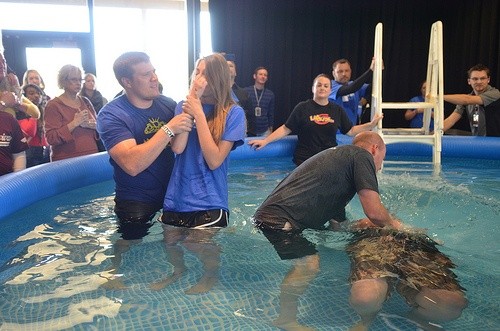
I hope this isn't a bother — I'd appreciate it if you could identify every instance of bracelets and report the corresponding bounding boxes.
[415,108,419,115]
[160,124,176,141]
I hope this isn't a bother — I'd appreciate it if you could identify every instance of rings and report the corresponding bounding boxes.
[88,120,91,125]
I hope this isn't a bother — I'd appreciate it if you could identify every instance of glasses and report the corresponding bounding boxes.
[64,78,85,82]
[469,76,489,81]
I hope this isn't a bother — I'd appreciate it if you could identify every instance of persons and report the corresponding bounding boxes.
[248,72,384,167]
[242,65,275,137]
[404,63,500,138]
[328,57,384,135]
[252,130,471,331]
[0,50,247,296]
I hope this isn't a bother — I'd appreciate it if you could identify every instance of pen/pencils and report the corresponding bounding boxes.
[78,108,81,113]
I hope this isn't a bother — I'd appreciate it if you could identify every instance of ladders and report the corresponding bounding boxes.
[370,21,444,174]
